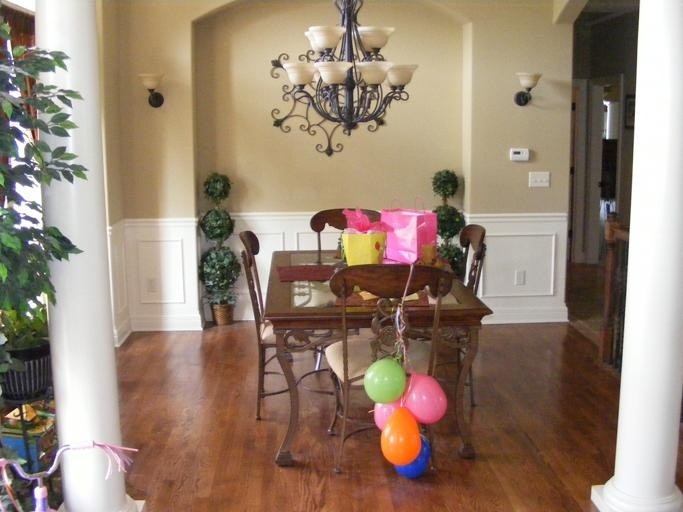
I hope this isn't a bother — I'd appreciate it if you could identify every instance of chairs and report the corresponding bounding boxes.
[235,226,341,423]
[401,223,487,410]
[320,259,454,479]
[308,208,385,376]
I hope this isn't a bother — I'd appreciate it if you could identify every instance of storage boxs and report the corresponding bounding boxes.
[0,414,59,484]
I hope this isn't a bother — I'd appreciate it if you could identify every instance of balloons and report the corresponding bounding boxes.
[363,357,447,477]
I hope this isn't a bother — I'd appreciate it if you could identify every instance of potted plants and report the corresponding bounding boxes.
[0,15,92,406]
[195,168,240,326]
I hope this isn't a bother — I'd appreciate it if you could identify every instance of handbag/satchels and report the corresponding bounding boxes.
[377,194,440,264]
[341,226,388,267]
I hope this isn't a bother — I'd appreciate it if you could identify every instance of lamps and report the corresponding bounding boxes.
[135,69,167,111]
[509,70,544,107]
[277,1,426,138]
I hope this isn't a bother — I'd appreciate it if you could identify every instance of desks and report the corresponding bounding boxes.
[261,245,494,470]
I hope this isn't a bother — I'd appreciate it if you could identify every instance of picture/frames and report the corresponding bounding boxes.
[622,92,635,130]
